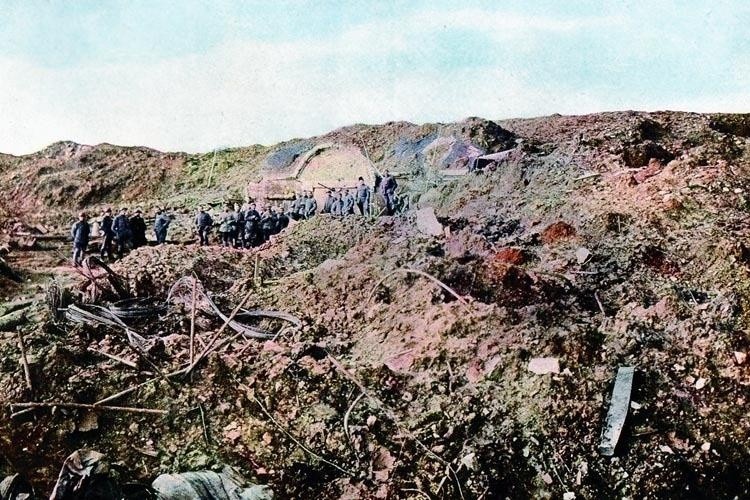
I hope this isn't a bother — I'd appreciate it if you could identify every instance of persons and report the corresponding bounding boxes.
[379,169,398,216]
[154,206,170,244]
[98,207,148,263]
[193,177,371,250]
[70,212,90,267]
